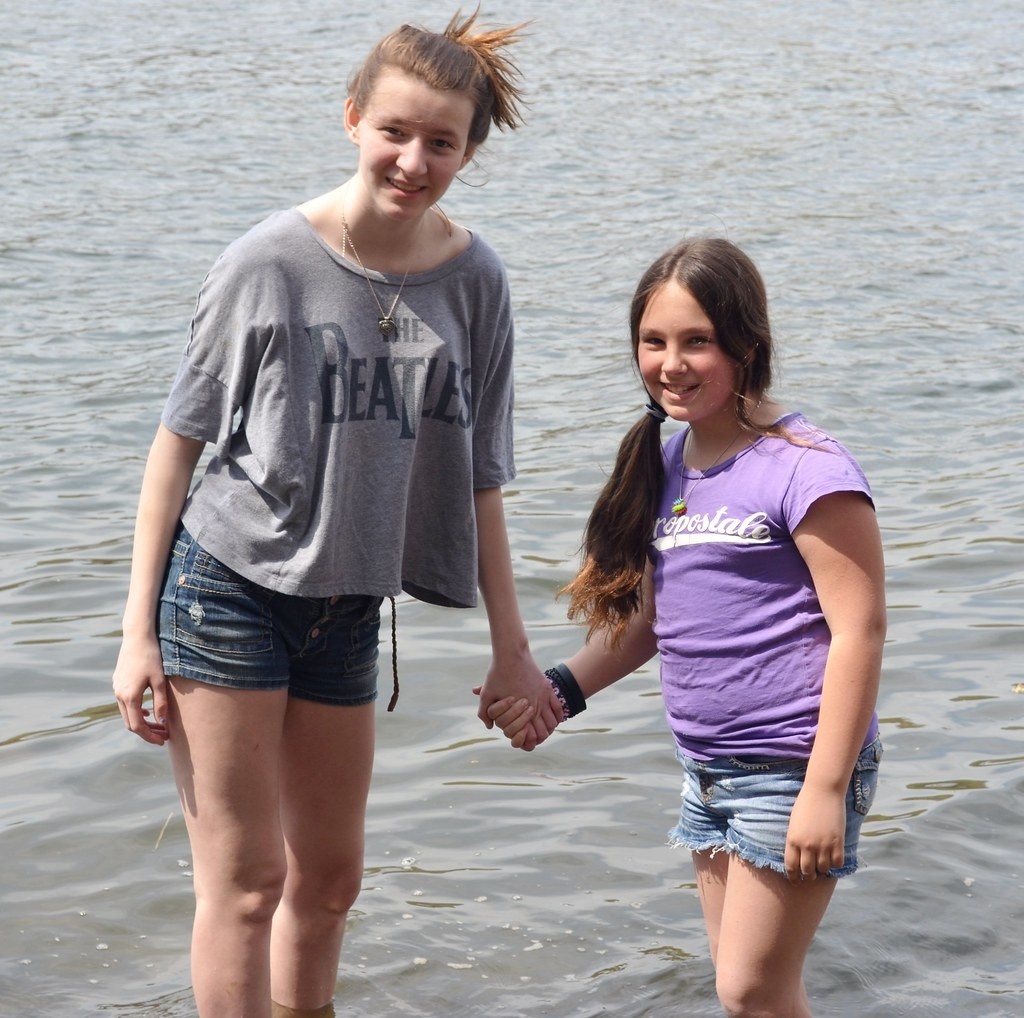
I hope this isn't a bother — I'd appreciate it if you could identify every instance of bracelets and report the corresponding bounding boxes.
[542,664,587,721]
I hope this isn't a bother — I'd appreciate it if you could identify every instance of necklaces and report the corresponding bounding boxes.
[339,185,427,336]
[670,398,765,514]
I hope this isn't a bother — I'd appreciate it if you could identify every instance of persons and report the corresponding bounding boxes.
[473,234,888,1017]
[112,9,565,1018]
